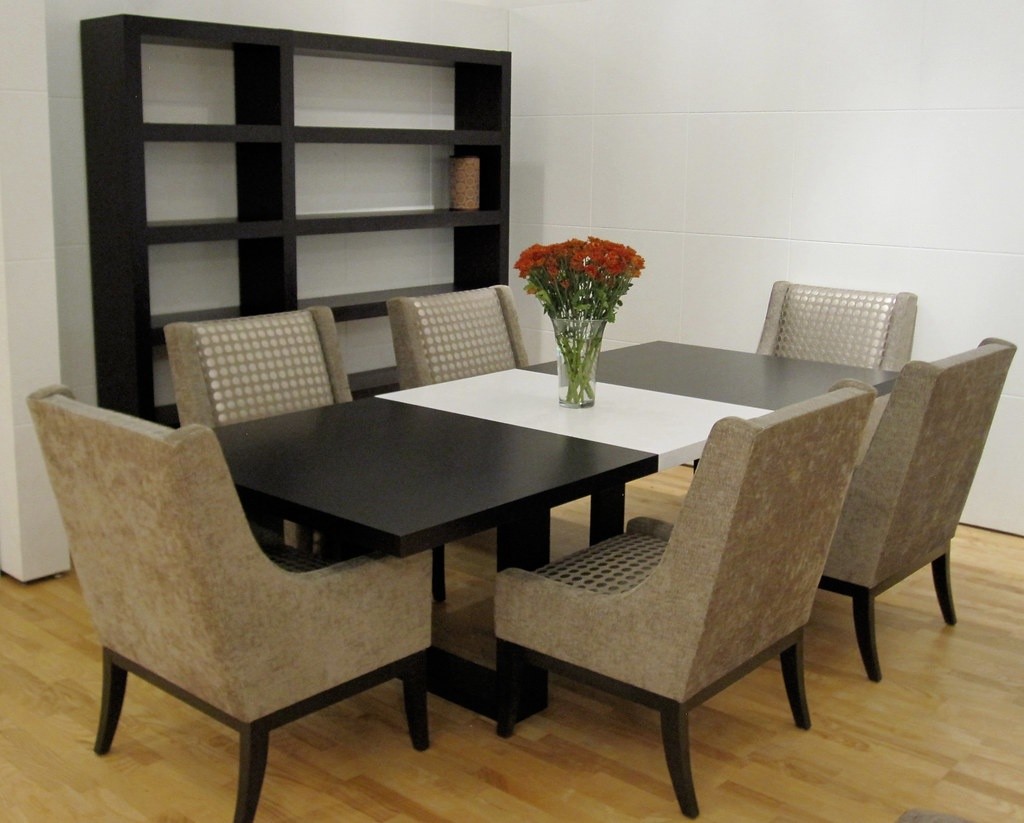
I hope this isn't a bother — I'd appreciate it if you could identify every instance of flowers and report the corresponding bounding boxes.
[513,235,646,404]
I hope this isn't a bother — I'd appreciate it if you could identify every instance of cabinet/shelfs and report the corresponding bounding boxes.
[79,13,513,431]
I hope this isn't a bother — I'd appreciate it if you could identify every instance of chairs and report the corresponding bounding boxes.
[818,338,1017,684]
[25,385,434,823]
[492,379,880,820]
[754,280,919,466]
[386,285,533,603]
[162,306,358,573]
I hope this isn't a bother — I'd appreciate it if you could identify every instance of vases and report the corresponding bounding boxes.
[549,318,611,408]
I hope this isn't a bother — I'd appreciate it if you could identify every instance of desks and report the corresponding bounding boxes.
[213,343,908,728]
